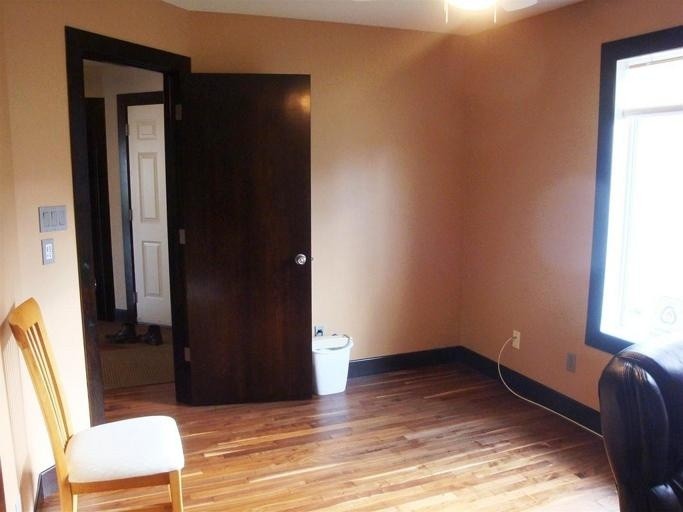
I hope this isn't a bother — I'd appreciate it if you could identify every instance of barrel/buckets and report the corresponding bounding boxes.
[312,334,354,395]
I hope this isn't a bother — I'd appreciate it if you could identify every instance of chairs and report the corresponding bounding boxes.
[7,297,185,512]
[598,339,683,512]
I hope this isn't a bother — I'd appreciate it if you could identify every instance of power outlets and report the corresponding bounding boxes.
[512,330,520,351]
[41,238,55,265]
